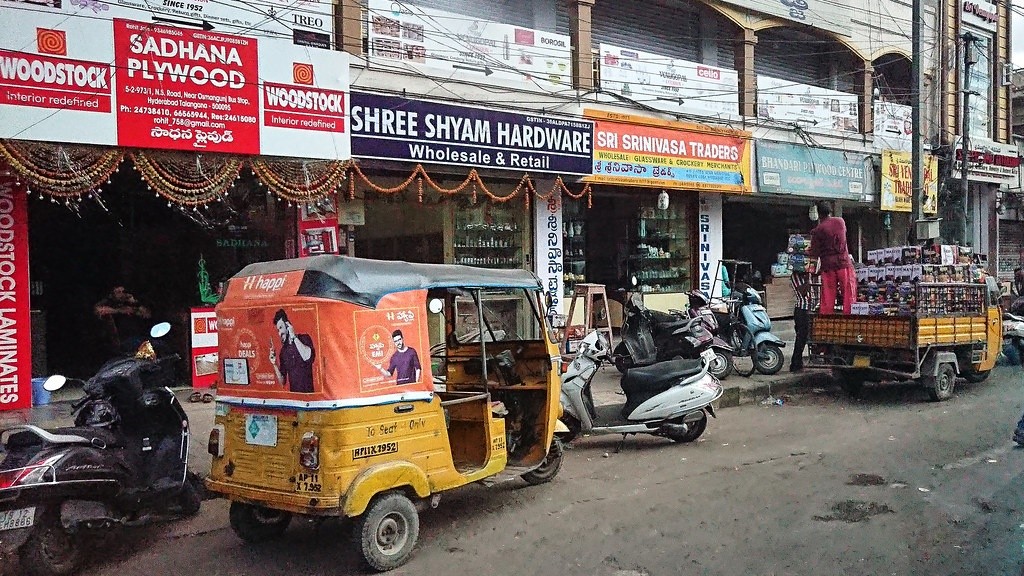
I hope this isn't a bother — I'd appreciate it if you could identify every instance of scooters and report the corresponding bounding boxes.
[553,328,725,453]
[607,273,789,381]
[0,319,203,576]
[1001,311,1024,364]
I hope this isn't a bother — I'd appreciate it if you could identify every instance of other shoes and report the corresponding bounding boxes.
[791,368,813,374]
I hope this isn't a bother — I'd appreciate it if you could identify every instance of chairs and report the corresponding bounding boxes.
[100,311,137,358]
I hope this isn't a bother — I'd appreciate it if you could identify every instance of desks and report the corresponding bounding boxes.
[558,282,614,356]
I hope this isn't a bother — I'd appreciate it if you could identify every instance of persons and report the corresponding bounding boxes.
[790,271,821,372]
[94,283,146,356]
[375,330,421,385]
[808,202,858,315]
[1012,268,1024,316]
[269,309,315,393]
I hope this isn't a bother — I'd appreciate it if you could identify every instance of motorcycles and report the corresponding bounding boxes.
[200,254,571,572]
[800,276,1007,401]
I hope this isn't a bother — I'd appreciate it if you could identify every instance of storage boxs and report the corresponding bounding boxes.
[847,244,986,317]
[770,233,822,277]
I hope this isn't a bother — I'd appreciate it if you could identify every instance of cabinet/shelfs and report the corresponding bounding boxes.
[637,200,689,295]
[561,185,587,297]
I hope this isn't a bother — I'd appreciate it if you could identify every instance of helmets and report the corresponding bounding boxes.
[75,400,121,435]
